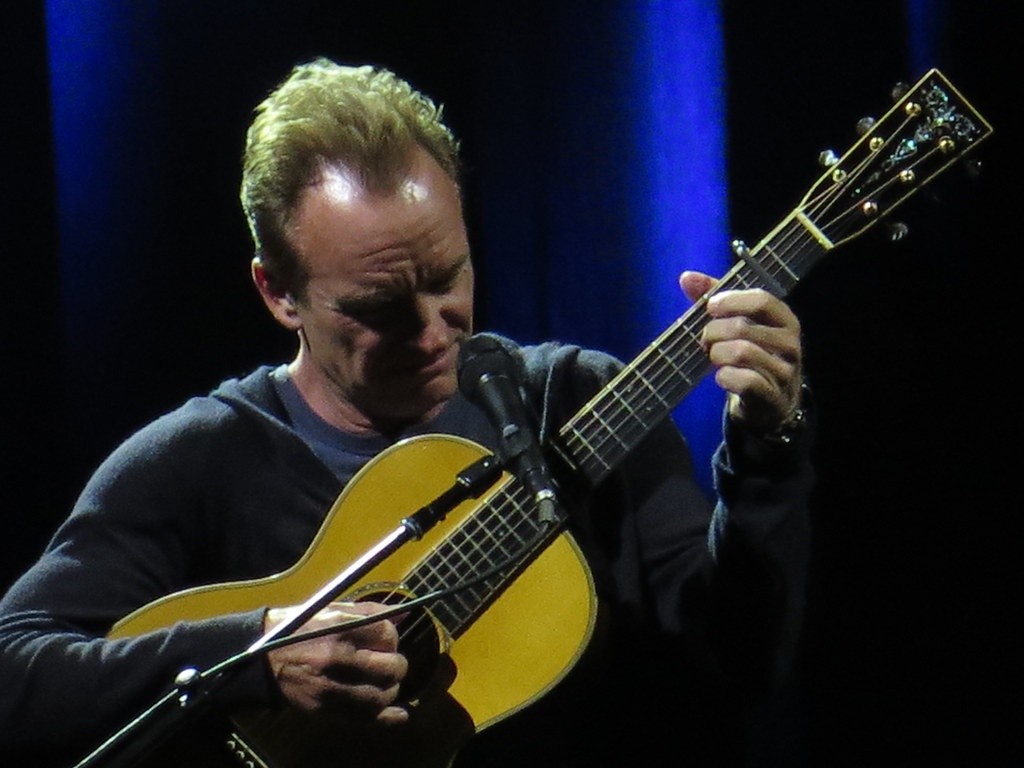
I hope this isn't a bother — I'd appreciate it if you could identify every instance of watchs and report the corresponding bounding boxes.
[763,385,814,445]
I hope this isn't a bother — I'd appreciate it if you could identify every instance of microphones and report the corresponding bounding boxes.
[457,334,556,523]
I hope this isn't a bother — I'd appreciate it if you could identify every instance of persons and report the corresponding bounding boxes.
[0,56,816,768]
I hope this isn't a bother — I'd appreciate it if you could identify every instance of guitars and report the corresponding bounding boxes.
[96,68,994,762]
